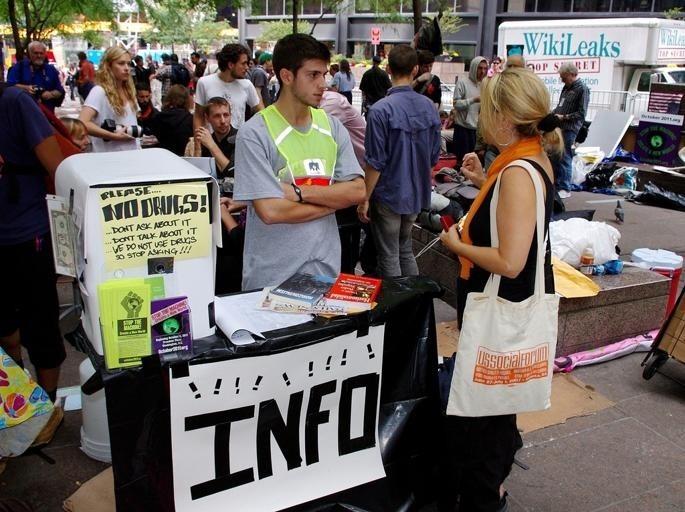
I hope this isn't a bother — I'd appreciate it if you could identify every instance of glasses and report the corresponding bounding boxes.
[492,61,498,64]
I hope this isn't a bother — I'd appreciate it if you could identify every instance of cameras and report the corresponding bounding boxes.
[101,119,144,142]
[33,85,44,95]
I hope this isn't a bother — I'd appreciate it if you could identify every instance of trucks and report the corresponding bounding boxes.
[496,16,685,124]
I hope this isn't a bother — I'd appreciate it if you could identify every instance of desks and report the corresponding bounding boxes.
[104,275,442,512]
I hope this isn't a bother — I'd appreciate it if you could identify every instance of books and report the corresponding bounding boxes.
[268,272,337,308]
[326,273,383,310]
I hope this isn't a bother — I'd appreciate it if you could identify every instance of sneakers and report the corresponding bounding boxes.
[28,404,65,449]
[557,189,572,199]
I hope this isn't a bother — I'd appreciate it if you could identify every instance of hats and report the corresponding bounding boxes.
[258,53,272,65]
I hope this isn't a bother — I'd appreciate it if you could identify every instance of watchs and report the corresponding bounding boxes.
[291,184,303,202]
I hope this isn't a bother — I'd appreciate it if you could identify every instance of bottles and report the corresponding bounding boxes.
[580,247,595,278]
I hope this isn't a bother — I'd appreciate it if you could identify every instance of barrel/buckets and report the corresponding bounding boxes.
[79,359,113,465]
[630,245,683,323]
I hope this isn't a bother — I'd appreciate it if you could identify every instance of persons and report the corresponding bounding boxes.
[0,38,280,448]
[440,67,566,512]
[356,44,442,276]
[233,33,367,292]
[316,54,590,199]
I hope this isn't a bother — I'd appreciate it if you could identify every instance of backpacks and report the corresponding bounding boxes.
[164,63,191,88]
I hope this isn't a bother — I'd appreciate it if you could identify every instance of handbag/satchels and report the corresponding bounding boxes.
[443,160,560,418]
[574,119,591,143]
